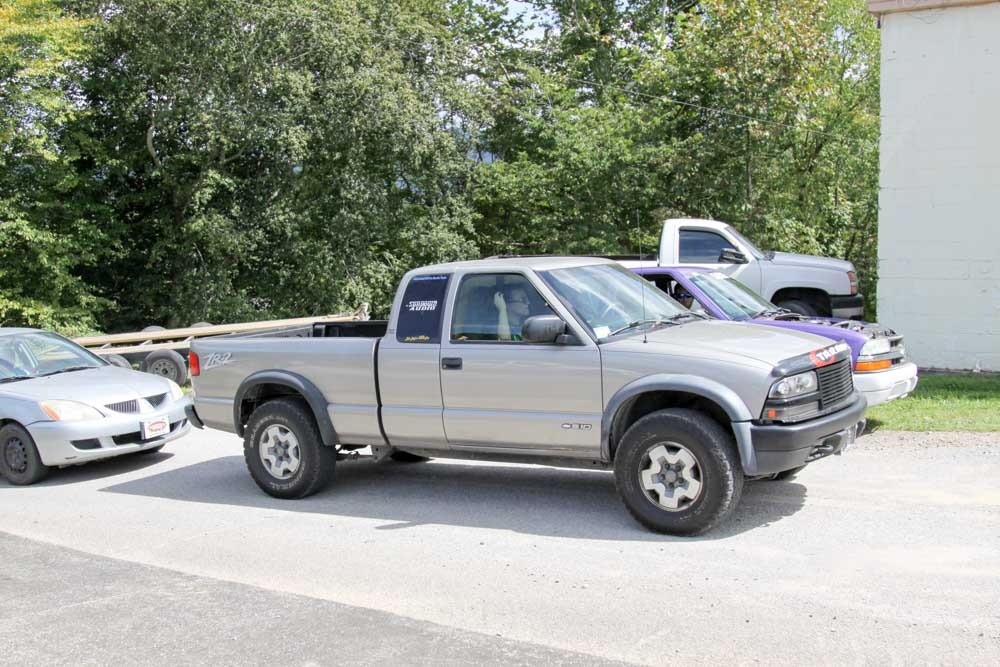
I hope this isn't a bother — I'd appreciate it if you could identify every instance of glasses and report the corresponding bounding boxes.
[675,292,693,298]
[507,297,529,303]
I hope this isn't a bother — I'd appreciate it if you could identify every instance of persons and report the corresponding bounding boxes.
[493,285,531,342]
[674,280,693,311]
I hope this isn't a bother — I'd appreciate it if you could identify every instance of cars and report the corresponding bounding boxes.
[0,327,191,486]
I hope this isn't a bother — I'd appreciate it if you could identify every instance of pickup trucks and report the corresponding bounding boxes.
[412,218,918,406]
[184,256,869,538]
